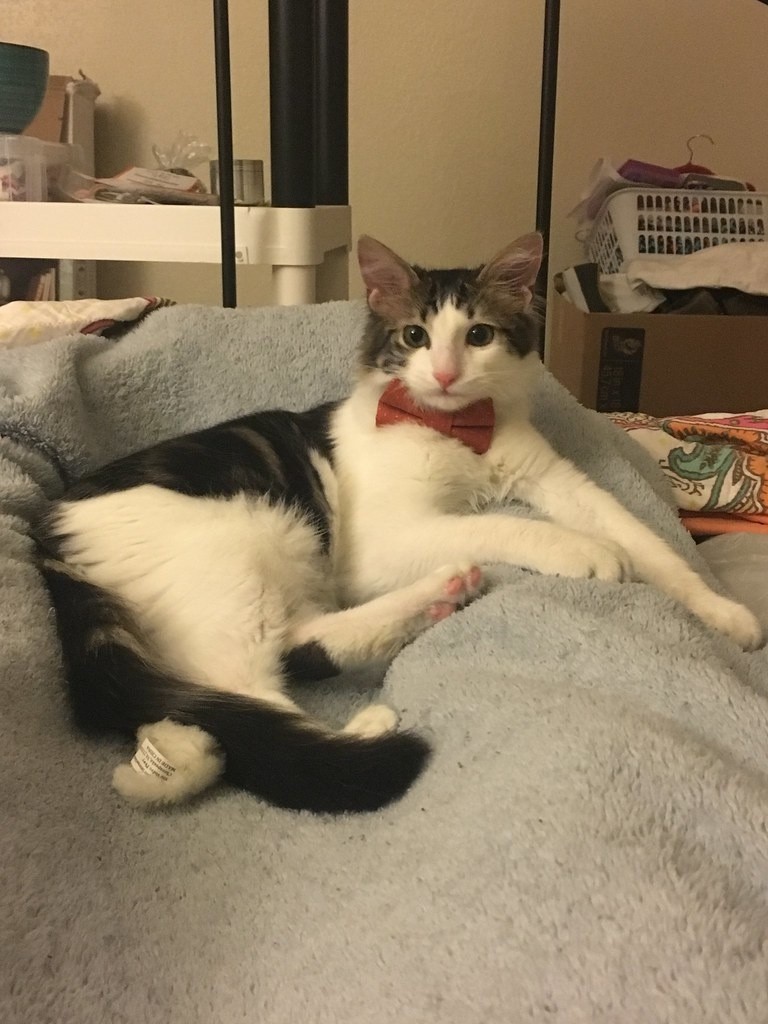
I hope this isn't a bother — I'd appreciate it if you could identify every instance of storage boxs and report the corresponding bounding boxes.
[576,186,768,278]
[549,263,767,423]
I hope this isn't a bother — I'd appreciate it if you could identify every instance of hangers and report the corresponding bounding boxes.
[622,132,724,189]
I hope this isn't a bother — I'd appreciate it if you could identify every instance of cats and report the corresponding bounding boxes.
[27,225,766,817]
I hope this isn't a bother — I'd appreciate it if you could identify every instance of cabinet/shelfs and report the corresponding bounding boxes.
[0,199,352,313]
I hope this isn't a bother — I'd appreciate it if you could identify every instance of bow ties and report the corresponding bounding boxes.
[373,381,494,457]
